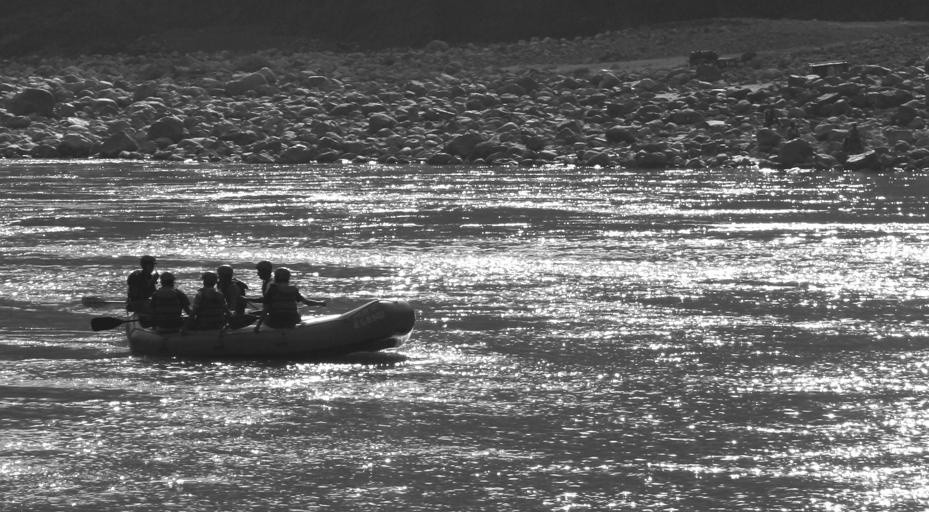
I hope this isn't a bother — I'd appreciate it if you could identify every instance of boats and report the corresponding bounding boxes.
[126,297,416,361]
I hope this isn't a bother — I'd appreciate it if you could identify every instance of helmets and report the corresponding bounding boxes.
[275,267,290,281]
[160,272,175,282]
[140,256,157,269]
[218,265,233,279]
[256,261,272,275]
[202,271,217,285]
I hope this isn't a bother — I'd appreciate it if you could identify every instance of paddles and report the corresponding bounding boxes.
[82,296,129,308]
[228,313,263,330]
[90,315,142,331]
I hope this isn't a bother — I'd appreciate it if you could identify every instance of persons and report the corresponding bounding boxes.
[186,271,226,330]
[241,261,275,315]
[216,265,245,314]
[253,267,327,334]
[126,255,159,315]
[149,272,192,336]
